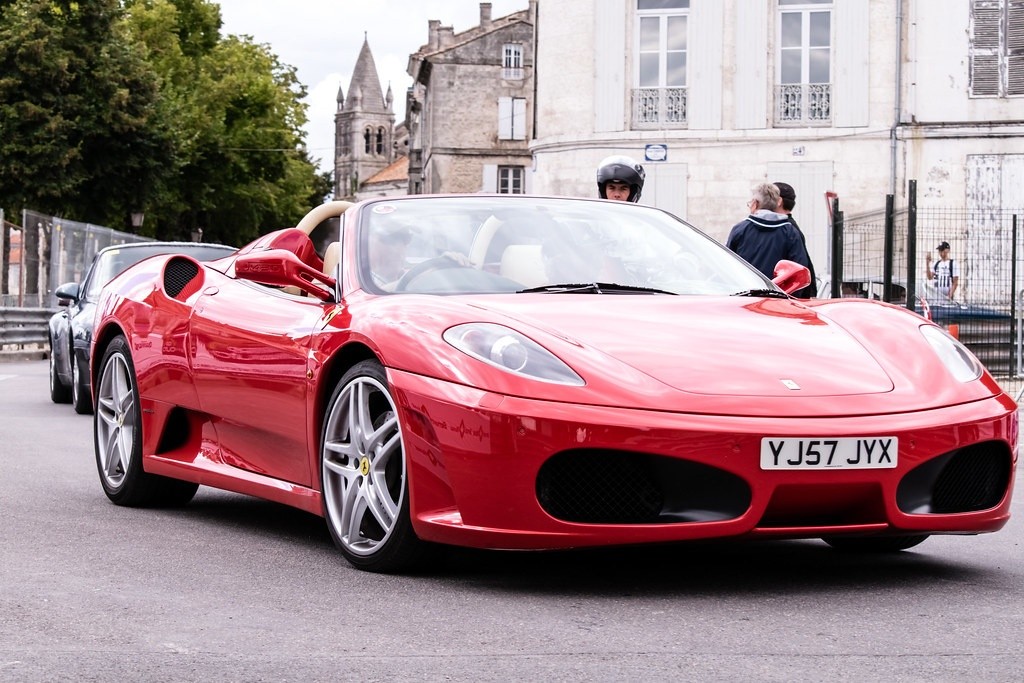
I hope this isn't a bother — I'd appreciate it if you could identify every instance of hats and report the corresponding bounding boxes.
[936,242,950,249]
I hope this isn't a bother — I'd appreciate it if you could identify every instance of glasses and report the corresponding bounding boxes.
[900,293,906,297]
[369,229,413,246]
[745,201,753,208]
[938,249,945,251]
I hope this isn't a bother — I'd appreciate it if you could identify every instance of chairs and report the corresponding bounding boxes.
[321,241,342,277]
[499,244,624,286]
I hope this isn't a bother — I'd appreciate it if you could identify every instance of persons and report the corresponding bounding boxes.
[924,241,960,300]
[325,208,479,297]
[596,163,646,203]
[724,181,819,299]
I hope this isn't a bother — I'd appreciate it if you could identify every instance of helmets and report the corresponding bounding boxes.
[597,164,646,203]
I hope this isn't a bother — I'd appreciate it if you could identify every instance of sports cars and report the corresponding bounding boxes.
[45,240,244,415]
[86,192,1019,573]
